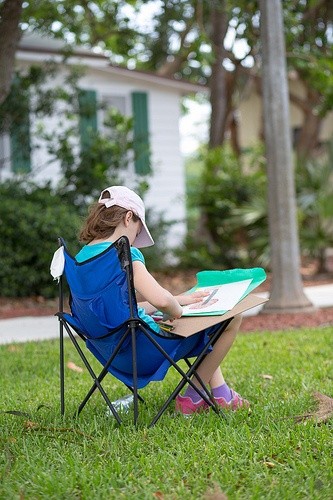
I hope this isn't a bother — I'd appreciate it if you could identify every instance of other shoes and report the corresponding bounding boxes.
[208,389,250,412]
[176,394,210,417]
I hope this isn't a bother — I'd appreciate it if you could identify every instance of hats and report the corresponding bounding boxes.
[97,186,154,248]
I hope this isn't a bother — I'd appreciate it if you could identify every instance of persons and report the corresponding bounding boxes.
[72,186,250,416]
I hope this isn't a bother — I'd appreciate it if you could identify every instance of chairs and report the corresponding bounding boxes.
[51,232,240,431]
